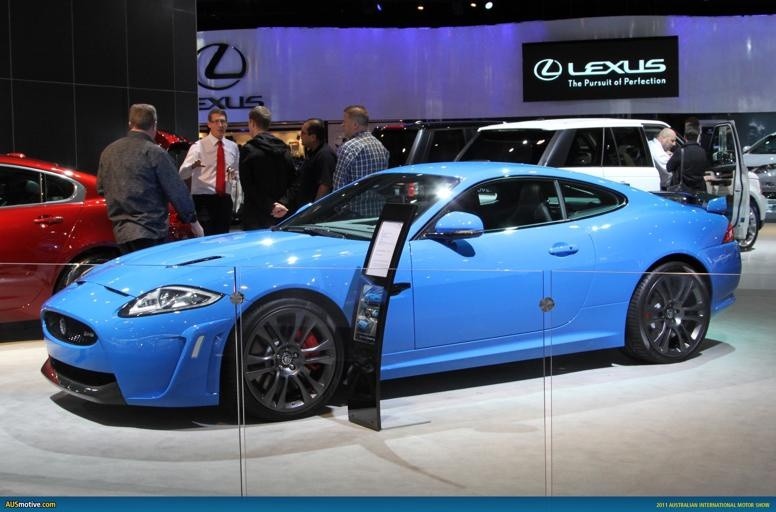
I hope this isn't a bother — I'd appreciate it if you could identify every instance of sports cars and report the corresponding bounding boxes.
[0,131,195,322]
[40,161,743,422]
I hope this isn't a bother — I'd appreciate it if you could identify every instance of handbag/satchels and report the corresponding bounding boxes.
[667,183,687,201]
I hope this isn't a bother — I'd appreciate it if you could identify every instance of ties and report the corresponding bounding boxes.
[216,140,225,196]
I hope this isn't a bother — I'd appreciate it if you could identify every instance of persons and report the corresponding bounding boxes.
[666,117,712,191]
[239,105,296,231]
[178,107,243,236]
[291,119,337,218]
[332,104,390,219]
[96,103,204,256]
[647,127,677,187]
